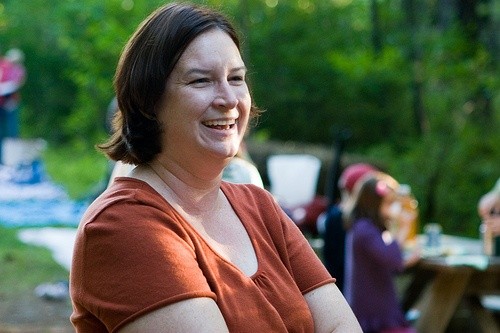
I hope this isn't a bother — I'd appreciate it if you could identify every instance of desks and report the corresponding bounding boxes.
[408,232,492,270]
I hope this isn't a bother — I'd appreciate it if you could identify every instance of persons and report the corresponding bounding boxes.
[69,3,363,332]
[0,46,27,162]
[323,159,500,332]
